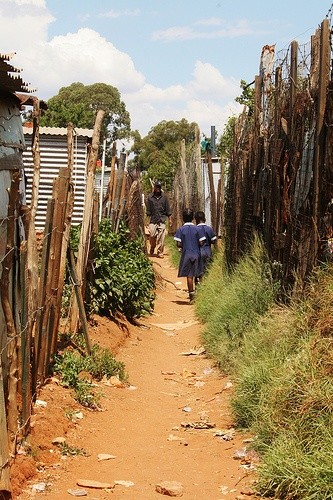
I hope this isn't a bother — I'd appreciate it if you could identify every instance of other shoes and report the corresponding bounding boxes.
[150,247,154,254]
[195,277,200,285]
[157,251,163,258]
[190,292,194,302]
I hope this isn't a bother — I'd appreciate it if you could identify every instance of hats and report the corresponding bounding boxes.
[155,181,162,187]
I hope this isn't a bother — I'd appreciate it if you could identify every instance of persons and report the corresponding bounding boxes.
[145,180,172,259]
[173,208,206,306]
[194,211,217,284]
